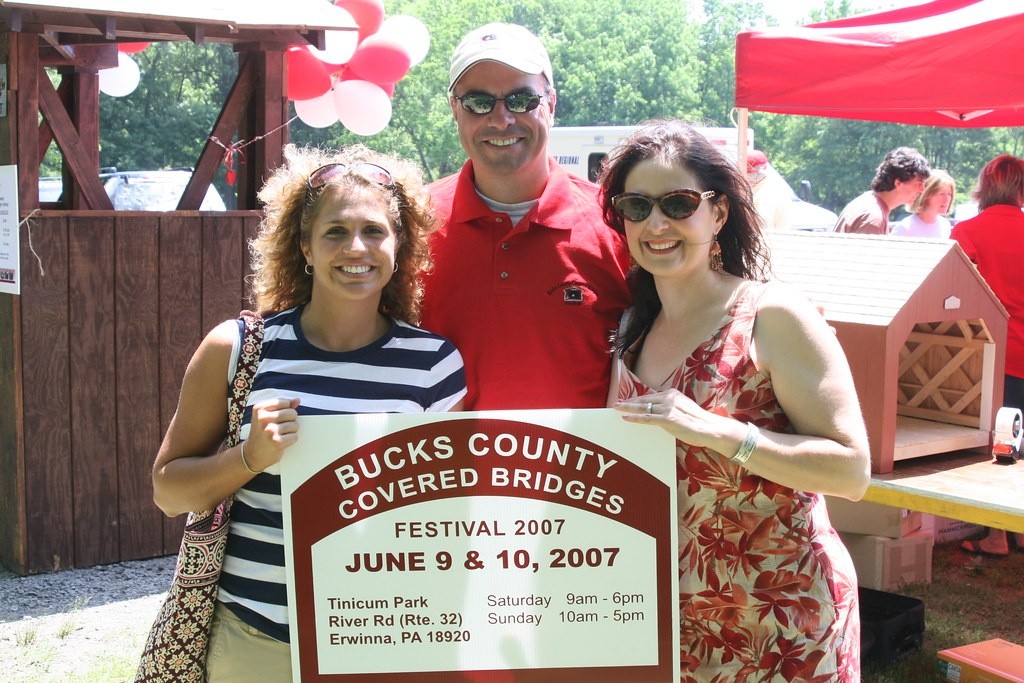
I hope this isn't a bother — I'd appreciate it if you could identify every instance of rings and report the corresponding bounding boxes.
[647,403,653,414]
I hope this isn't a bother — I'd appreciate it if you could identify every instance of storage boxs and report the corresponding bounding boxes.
[824,493,985,671]
[937,637,1024,683]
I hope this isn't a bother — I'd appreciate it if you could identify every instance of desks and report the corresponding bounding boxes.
[862,450,1024,535]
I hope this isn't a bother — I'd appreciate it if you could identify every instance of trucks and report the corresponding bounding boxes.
[547,127,839,233]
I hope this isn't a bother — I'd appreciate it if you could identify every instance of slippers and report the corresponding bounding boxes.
[959,540,1008,557]
[1013,535,1024,551]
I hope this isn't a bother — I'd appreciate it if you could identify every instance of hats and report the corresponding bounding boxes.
[449,20,554,89]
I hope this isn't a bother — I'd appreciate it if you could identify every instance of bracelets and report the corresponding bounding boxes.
[728,422,760,465]
[240,441,263,474]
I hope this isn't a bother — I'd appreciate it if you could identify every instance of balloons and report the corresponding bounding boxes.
[336,80,394,136]
[293,91,339,129]
[97,51,141,98]
[285,0,409,101]
[118,42,151,55]
[380,16,431,64]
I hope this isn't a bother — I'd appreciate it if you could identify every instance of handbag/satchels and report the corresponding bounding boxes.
[134,308,264,683]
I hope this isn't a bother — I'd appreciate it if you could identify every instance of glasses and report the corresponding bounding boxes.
[611,190,718,223]
[453,91,548,116]
[304,162,400,209]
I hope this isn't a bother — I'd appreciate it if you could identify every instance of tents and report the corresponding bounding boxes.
[733,1,1024,189]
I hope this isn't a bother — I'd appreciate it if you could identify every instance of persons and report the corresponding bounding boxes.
[402,22,637,411]
[833,146,931,235]
[947,153,1024,556]
[148,146,469,683]
[890,170,957,240]
[597,121,872,682]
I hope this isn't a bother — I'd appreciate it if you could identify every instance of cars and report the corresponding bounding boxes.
[57,165,227,211]
